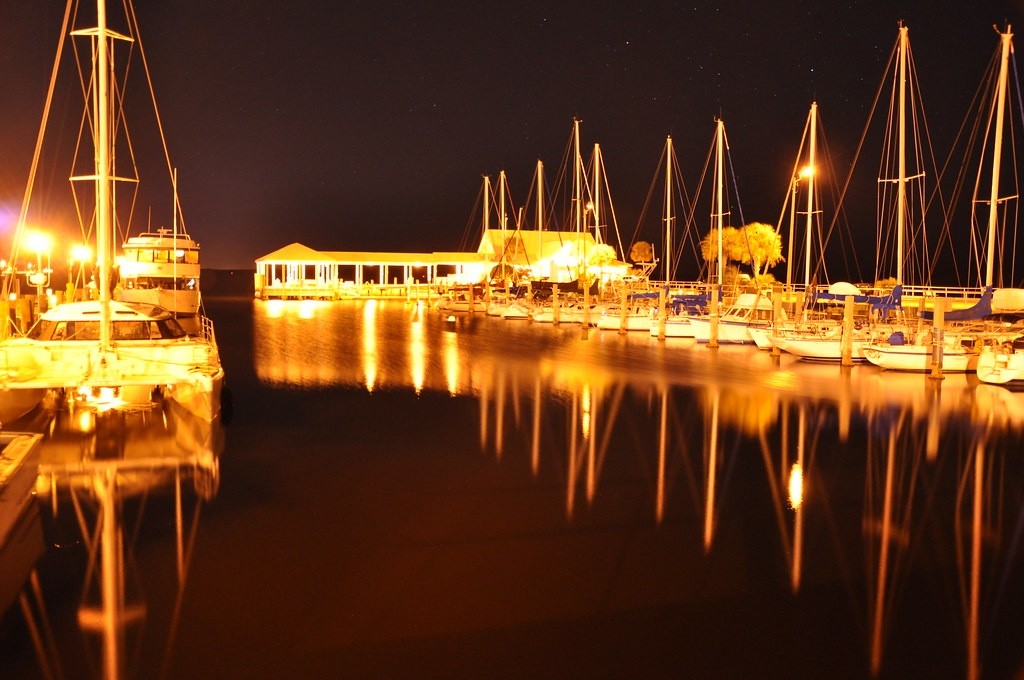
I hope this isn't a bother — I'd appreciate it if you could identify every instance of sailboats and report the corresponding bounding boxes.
[436,17,1023,387]
[1,1,225,613]
